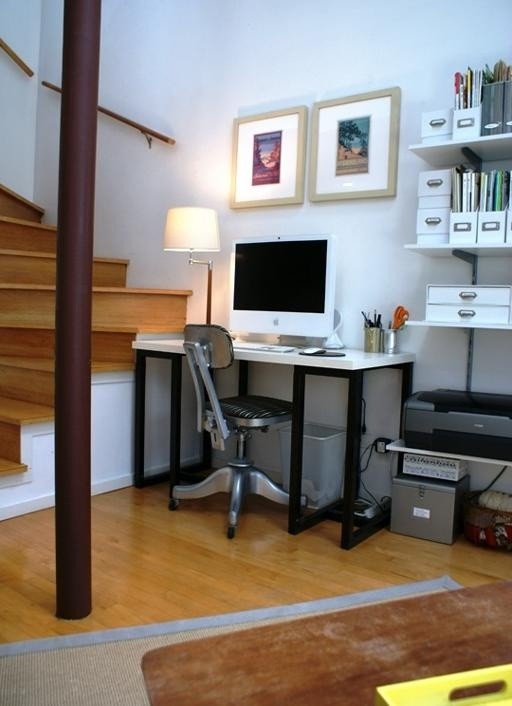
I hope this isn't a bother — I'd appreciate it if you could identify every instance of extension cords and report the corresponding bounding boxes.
[364,498,389,518]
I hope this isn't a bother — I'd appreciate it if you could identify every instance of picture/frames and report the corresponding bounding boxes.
[229,86,401,208]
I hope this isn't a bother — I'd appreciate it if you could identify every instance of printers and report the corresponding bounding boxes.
[402,387,512,464]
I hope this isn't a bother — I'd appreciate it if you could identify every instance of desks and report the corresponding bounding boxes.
[386,439,512,545]
[131,340,416,550]
[141,579,512,706]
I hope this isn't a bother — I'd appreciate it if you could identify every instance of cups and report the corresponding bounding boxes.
[364,327,399,354]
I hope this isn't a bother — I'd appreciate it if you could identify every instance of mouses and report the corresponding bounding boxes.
[299,348,327,354]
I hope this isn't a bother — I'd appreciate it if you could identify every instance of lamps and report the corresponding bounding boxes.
[163,207,221,324]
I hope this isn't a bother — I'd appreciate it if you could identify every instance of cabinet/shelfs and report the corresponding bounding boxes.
[403,132,512,330]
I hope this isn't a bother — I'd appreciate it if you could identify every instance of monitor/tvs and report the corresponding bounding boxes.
[229,232,335,346]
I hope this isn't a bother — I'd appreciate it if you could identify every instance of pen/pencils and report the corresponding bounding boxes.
[454,60,512,110]
[361,309,382,327]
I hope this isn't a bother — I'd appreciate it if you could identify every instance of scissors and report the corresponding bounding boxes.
[392,305,409,329]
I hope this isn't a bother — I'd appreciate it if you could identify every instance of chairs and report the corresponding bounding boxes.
[169,324,308,538]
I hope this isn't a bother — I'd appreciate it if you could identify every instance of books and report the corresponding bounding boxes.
[447,57,512,213]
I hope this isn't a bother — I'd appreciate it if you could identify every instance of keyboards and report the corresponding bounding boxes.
[233,343,294,352]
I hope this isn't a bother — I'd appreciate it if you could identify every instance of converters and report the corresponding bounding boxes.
[374,437,391,453]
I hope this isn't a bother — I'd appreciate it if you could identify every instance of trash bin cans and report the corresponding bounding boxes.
[277,422,347,510]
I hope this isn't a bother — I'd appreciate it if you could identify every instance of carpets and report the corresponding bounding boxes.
[0,574,463,706]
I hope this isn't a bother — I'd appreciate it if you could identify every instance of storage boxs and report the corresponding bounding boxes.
[390,474,470,545]
